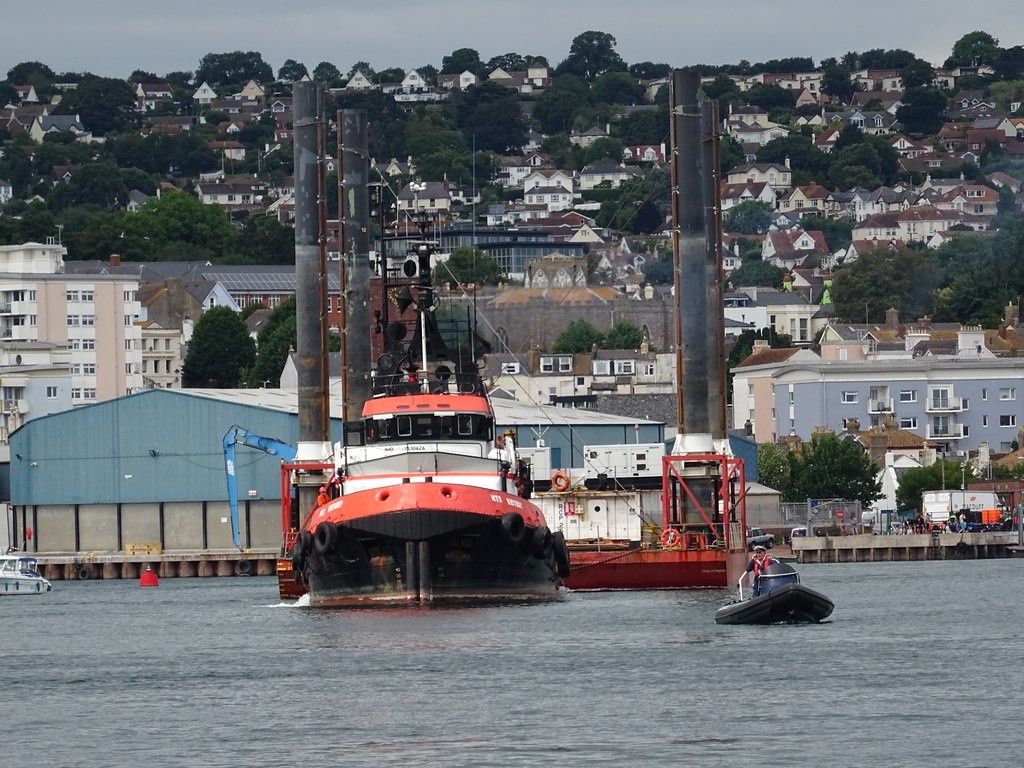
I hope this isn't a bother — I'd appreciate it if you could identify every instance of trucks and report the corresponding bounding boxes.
[920,489,1006,532]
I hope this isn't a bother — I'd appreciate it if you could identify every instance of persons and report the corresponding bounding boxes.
[318,487,330,508]
[787,501,1023,557]
[746,548,773,598]
[512,459,532,503]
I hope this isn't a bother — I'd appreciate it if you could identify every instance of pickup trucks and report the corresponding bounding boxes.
[746,527,775,551]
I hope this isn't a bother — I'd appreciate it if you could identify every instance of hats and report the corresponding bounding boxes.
[753,546,766,554]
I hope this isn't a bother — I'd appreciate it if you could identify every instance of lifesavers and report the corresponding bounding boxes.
[661,528,680,547]
[552,471,570,492]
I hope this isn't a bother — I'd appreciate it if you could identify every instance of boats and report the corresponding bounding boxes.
[0,526,52,596]
[277,70,757,601]
[715,554,835,626]
[288,133,574,610]
